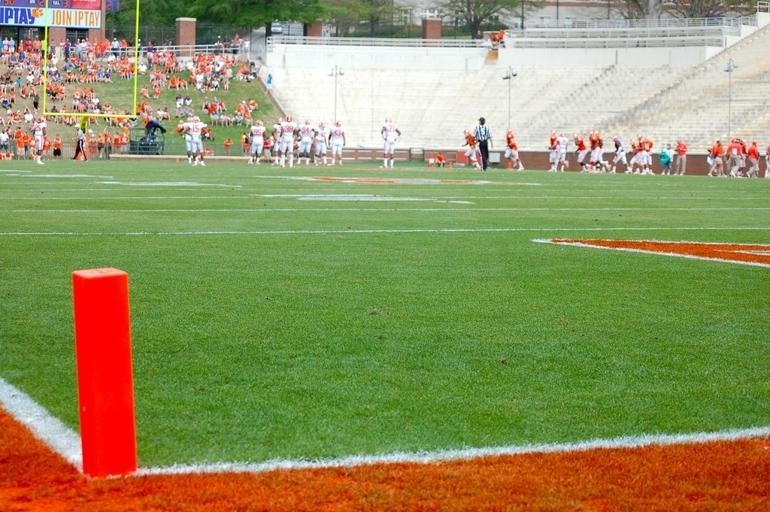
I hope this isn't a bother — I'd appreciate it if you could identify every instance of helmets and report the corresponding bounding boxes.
[184,114,396,130]
[35,114,46,123]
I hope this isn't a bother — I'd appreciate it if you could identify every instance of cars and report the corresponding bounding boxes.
[271,22,283,33]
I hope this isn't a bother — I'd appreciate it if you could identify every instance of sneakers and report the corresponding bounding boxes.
[35,156,45,165]
[187,157,396,170]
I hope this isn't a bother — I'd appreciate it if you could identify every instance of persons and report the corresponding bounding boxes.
[379,116,401,169]
[461,129,481,170]
[504,128,514,170]
[497,27,506,50]
[546,128,770,180]
[507,133,524,172]
[706,8,718,21]
[489,32,500,50]
[434,151,446,168]
[471,117,494,172]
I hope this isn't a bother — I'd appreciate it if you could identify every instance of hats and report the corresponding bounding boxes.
[477,116,485,121]
[75,123,80,127]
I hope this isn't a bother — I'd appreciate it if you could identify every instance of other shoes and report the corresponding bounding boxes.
[549,168,685,177]
[709,170,769,179]
[517,167,525,172]
[474,165,487,174]
[513,163,518,168]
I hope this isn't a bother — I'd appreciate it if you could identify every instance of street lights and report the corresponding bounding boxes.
[327,62,344,123]
[723,56,737,137]
[502,63,518,130]
